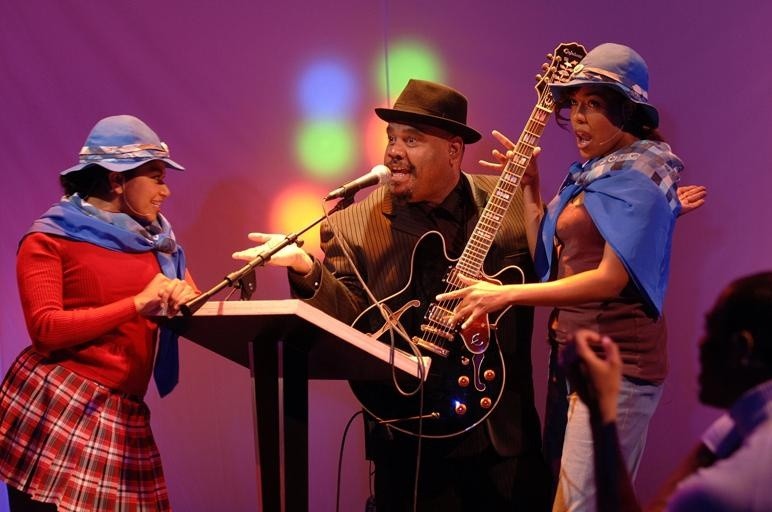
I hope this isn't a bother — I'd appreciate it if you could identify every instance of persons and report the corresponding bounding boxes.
[233,79,707,511]
[0,113,204,512]
[434,42,682,512]
[563,269,771,512]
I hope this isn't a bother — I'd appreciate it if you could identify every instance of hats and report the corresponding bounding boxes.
[61,115,186,177]
[548,43,660,127]
[375,79,482,144]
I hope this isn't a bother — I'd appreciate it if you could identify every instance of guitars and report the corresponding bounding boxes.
[345,42,589,438]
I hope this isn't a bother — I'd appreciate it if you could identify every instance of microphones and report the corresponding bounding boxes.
[325,164,391,200]
